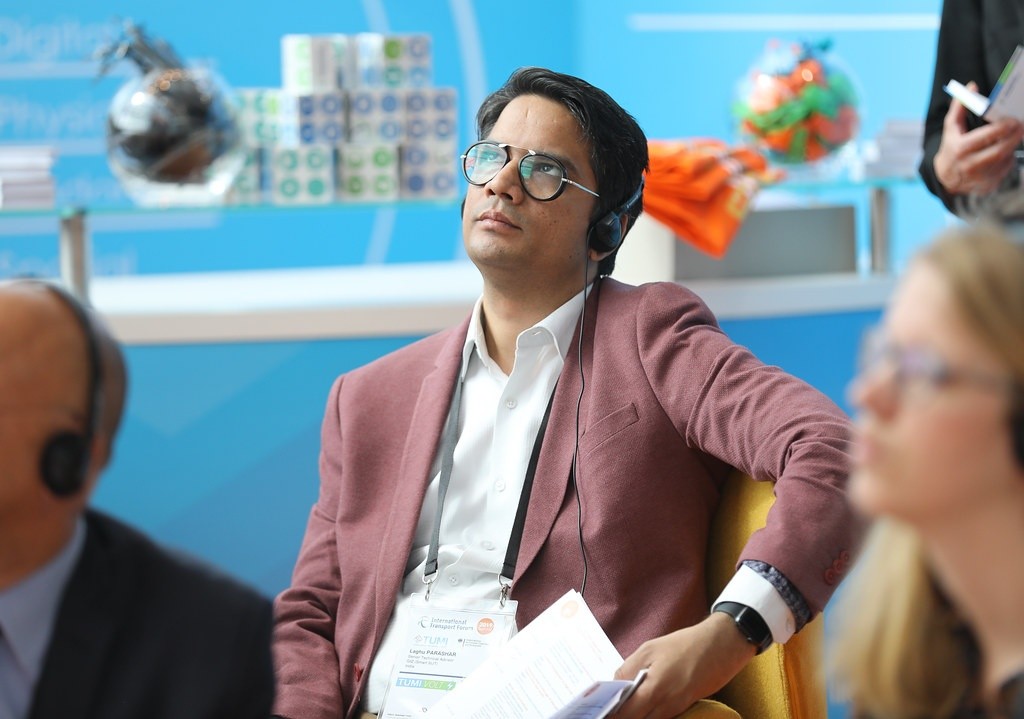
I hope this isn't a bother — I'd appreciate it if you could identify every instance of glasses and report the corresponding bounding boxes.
[858,328,1024,391]
[461,140,618,207]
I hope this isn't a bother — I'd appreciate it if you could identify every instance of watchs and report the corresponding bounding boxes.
[713,602,773,656]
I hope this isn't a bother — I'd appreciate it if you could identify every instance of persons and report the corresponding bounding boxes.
[836,220,1024,719]
[0,277,278,719]
[917,0,1024,223]
[269,68,876,719]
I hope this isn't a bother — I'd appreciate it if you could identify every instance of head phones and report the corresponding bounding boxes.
[460,170,647,254]
[28,277,109,493]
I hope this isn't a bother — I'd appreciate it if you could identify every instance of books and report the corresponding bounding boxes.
[944,45,1024,126]
